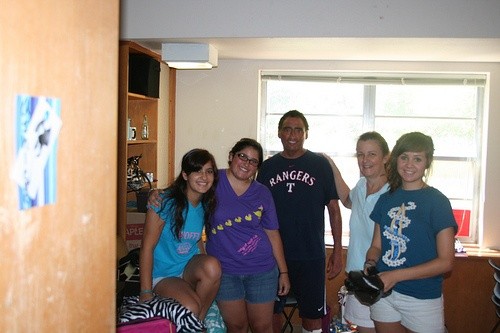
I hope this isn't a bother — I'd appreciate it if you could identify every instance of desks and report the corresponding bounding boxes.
[272,245,500,333]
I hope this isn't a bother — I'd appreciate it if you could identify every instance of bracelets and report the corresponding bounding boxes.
[140,289,152,294]
[365,263,373,266]
[279,272,290,274]
[366,259,375,261]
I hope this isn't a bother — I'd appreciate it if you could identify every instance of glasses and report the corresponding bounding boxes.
[237,153,259,167]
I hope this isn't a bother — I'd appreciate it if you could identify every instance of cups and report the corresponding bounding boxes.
[128,118,136,141]
[145,172,153,182]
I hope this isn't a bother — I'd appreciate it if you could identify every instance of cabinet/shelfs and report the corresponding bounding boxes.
[116,38,176,242]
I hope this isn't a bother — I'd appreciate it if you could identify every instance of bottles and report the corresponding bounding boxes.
[142,114,149,140]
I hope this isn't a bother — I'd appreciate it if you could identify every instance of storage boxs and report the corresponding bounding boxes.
[126,208,146,251]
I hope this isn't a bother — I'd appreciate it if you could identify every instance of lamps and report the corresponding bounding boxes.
[162,42,219,69]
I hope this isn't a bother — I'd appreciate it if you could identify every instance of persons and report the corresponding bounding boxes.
[364,132,458,333]
[140,148,222,327]
[148,138,291,333]
[318,131,390,333]
[255,110,344,333]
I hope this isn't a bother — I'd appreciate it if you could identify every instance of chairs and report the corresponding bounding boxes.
[488,259,500,318]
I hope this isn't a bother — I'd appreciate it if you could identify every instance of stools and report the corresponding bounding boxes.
[281,296,298,333]
